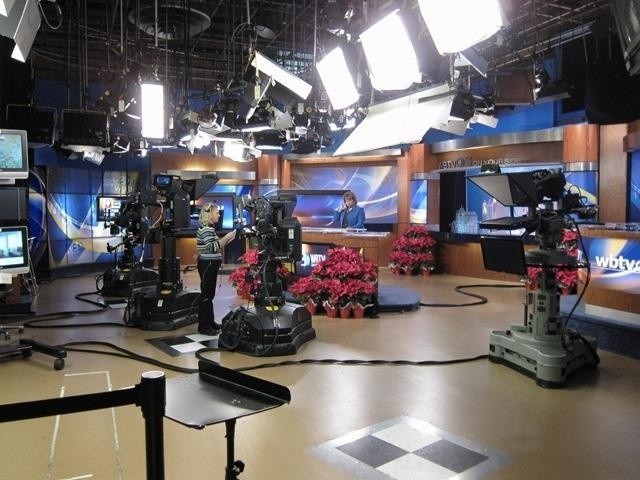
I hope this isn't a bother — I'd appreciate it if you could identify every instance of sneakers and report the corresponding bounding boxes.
[198,325,220,336]
[211,321,223,330]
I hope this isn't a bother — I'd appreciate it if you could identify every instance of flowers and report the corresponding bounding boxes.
[227,248,290,302]
[388,226,438,278]
[287,245,380,319]
[525,231,580,295]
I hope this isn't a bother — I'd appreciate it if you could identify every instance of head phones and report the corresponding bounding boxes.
[206,204,213,212]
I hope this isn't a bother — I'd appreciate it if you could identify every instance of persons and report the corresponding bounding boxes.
[332,189,365,229]
[195,201,238,337]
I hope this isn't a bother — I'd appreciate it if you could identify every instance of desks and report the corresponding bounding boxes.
[301,225,392,269]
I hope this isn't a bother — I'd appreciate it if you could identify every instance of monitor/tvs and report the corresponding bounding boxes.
[0,129,29,185]
[480,236,527,276]
[0,226,30,278]
